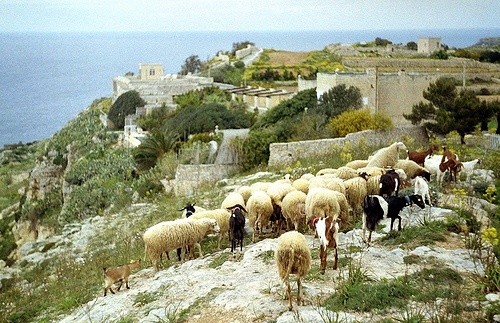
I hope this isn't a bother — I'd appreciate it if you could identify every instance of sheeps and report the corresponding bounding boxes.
[101,257,142,296]
[141,140,479,275]
[274,229,314,312]
